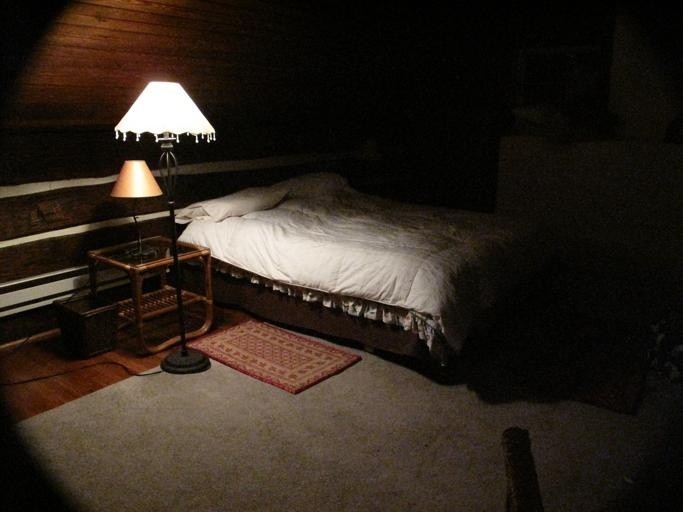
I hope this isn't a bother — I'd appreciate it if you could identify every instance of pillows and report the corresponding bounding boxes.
[181,171,347,223]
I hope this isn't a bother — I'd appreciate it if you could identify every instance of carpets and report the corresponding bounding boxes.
[0,317,683,512]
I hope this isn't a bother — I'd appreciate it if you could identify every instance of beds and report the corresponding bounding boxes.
[177,187,536,374]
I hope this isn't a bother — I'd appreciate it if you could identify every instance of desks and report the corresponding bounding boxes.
[83,236,209,356]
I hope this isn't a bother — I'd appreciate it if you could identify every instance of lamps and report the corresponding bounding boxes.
[109,73,224,374]
